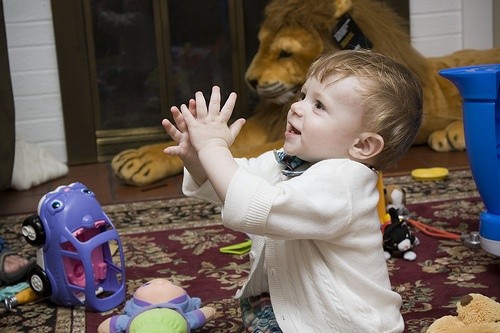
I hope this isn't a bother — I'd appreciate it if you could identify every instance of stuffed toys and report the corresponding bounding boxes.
[112,0,500,186]
[380,185,421,261]
[97,278,217,333]
[426,293,500,333]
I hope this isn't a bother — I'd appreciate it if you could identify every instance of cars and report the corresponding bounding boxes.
[22,182,126,310]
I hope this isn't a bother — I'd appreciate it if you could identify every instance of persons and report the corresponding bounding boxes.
[162,50,423,333]
[1,239,37,285]
